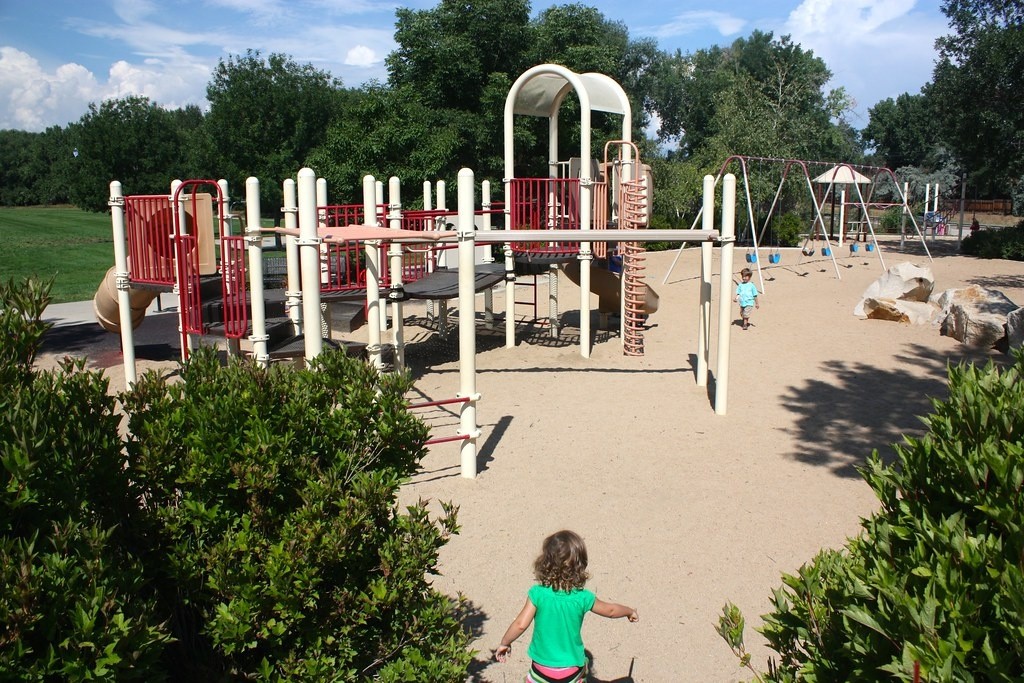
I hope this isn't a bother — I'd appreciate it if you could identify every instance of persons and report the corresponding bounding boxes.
[734,268,759,330]
[495,530,639,683]
[971,218,980,231]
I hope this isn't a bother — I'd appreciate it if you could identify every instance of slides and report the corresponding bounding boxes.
[560,262,660,315]
[92,255,162,335]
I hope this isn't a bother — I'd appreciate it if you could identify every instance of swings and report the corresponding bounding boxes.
[746,156,763,263]
[802,160,819,257]
[821,162,837,257]
[865,166,880,251]
[850,164,864,253]
[768,158,785,264]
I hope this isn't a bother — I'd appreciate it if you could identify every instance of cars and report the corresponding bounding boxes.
[915,211,947,229]
[230,200,247,215]
